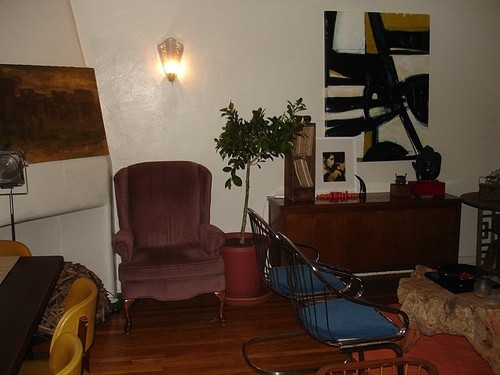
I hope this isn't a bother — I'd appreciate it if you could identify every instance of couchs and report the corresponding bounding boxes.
[111,160,226,335]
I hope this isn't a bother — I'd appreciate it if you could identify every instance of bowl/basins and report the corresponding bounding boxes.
[438,263,479,284]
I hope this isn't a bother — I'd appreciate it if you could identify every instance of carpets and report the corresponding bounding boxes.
[352,304,490,373]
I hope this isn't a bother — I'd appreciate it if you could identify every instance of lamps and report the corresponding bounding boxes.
[157,37,184,82]
[0,148,30,240]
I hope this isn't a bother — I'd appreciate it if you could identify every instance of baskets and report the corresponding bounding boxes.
[38,262,113,339]
[478,175,500,201]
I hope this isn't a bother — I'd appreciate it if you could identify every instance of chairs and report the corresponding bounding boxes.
[16,334,83,375]
[29,277,98,373]
[242,208,363,373]
[278,231,409,375]
[0,241,30,256]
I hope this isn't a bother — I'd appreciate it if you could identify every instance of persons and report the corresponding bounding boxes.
[323,153,345,182]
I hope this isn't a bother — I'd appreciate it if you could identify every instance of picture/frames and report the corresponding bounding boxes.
[315,138,359,197]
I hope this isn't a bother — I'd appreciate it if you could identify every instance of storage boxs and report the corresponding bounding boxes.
[408,181,446,197]
[391,184,409,197]
[285,121,315,201]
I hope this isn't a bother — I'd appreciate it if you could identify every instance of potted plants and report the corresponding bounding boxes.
[214,98,312,305]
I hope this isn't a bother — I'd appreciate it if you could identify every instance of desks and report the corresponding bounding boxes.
[1,256,64,375]
[397,265,500,374]
[267,192,461,274]
[460,193,500,268]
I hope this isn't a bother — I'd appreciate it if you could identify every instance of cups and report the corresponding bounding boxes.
[475,277,491,296]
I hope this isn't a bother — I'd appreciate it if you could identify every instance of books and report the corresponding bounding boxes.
[292,127,314,156]
[294,159,313,188]
